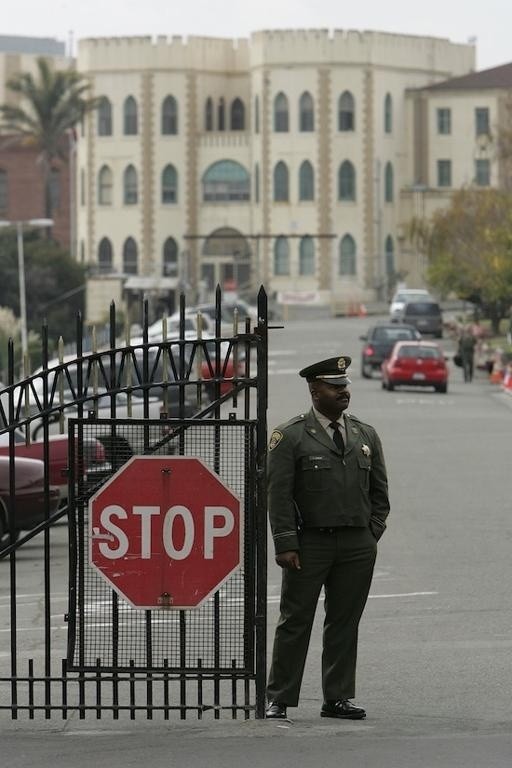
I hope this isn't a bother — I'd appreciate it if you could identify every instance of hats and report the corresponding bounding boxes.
[298,356,352,388]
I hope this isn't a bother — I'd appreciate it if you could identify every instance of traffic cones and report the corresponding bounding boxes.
[489,353,504,384]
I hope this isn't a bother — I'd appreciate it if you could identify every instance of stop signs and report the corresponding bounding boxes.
[88,455,243,611]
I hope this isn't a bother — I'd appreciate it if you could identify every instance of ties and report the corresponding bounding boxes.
[330,422,345,455]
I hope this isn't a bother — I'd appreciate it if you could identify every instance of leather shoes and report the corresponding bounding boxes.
[320,699,366,719]
[264,700,287,719]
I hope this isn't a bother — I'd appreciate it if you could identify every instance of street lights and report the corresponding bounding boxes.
[0,218,56,379]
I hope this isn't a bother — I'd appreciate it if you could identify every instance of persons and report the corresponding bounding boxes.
[263,354,392,720]
[458,325,478,382]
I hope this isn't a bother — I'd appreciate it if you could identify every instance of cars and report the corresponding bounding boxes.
[361,288,450,392]
